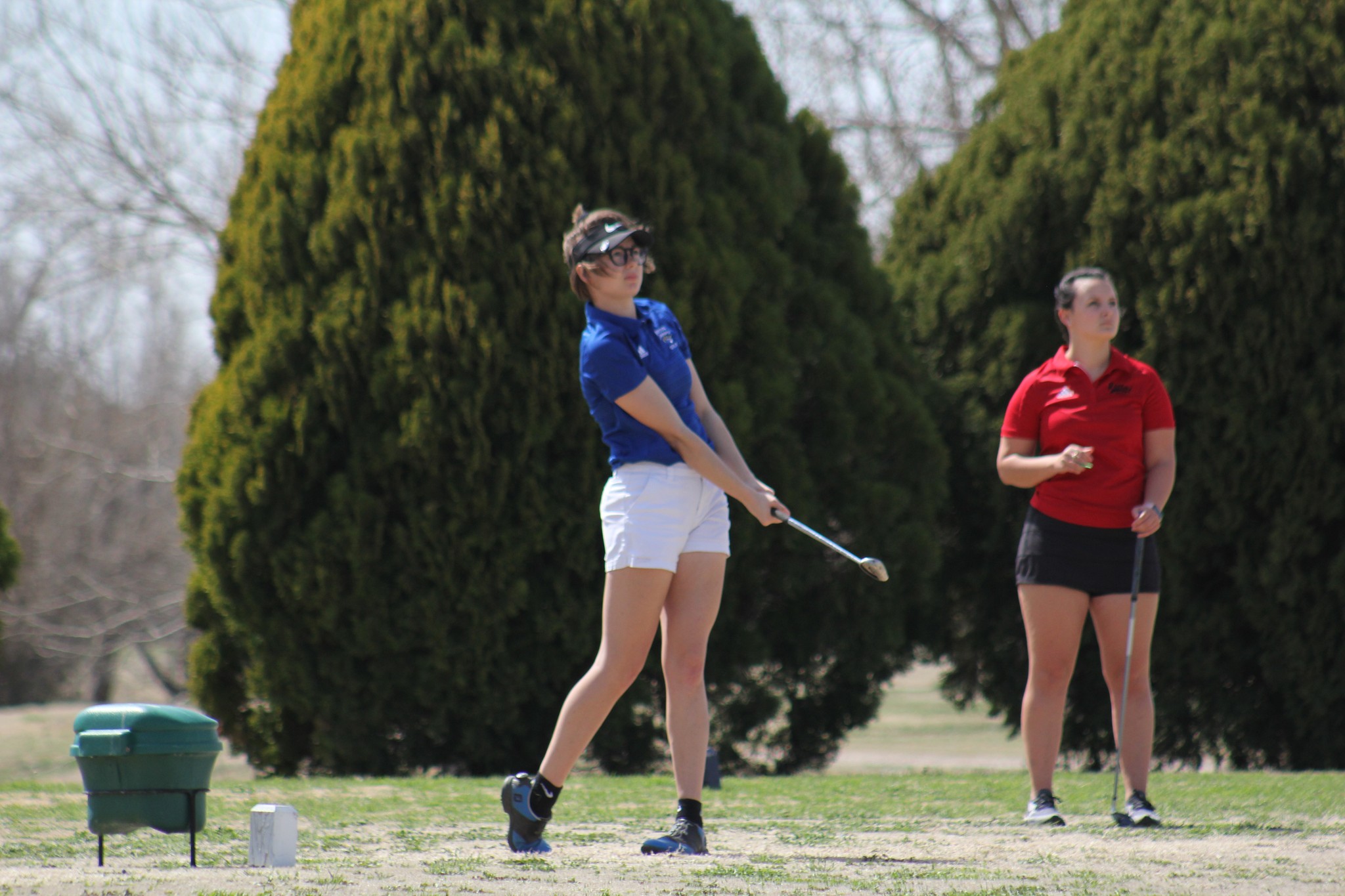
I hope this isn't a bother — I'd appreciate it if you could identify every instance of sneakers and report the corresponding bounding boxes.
[1025,788,1067,826]
[501,773,552,853]
[1125,789,1161,826]
[641,818,708,854]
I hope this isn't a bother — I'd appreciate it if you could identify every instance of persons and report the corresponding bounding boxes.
[997,265,1177,828]
[502,203,790,855]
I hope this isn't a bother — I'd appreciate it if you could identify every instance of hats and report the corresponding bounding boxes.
[568,219,650,265]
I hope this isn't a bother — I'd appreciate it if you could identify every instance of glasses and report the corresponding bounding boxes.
[587,244,646,265]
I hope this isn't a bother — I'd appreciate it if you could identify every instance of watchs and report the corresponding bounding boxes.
[1145,503,1164,521]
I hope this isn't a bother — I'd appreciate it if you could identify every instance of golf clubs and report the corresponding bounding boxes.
[1111,511,1143,827]
[770,506,889,582]
